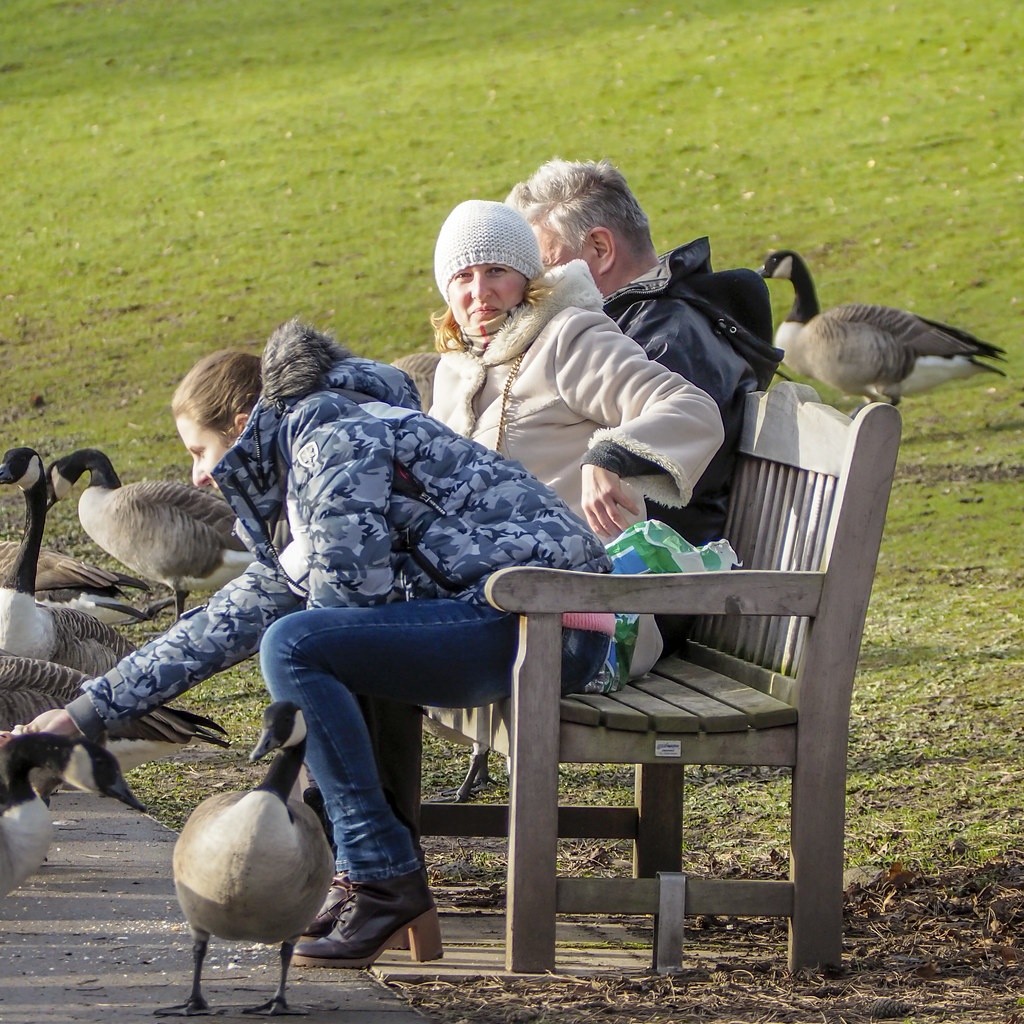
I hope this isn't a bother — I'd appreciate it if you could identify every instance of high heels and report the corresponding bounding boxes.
[289,867,444,969]
[299,870,411,950]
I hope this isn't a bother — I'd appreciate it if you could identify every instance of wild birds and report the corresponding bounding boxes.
[0,656,233,808]
[753,250,1010,420]
[0,447,140,680]
[421,711,500,803]
[152,702,340,1016]
[0,542,152,625]
[0,733,148,902]
[44,448,259,647]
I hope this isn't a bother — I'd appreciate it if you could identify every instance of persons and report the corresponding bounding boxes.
[492,153,787,655]
[13,323,618,968]
[370,198,726,886]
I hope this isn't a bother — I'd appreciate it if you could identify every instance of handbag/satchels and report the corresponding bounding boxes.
[572,519,744,694]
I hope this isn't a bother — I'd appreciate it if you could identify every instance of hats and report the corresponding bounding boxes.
[434,199,544,306]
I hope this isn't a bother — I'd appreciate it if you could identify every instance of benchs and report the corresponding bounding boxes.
[296,386,905,975]
